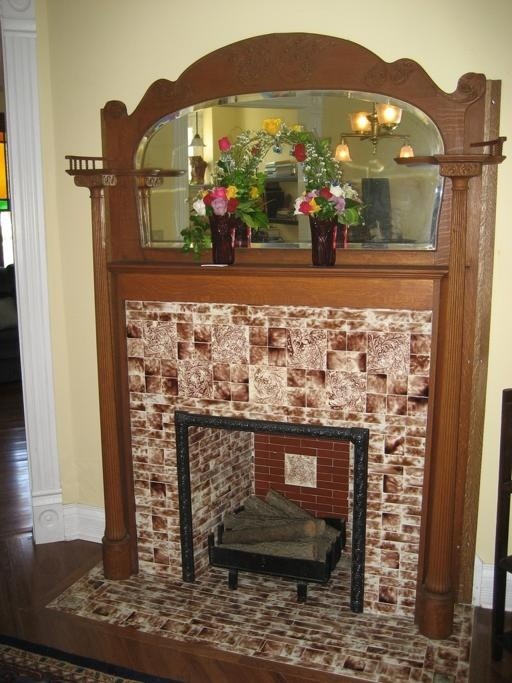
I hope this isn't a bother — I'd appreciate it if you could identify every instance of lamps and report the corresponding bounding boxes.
[334,100,415,163]
[190,112,207,146]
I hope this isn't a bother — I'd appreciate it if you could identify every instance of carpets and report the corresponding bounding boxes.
[0,633,193,683]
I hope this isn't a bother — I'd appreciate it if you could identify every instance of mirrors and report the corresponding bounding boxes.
[100,31,503,270]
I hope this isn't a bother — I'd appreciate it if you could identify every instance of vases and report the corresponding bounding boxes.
[234,216,253,248]
[309,212,338,267]
[208,213,237,266]
[336,221,347,249]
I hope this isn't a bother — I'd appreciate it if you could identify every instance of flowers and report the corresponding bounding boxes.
[338,182,368,228]
[278,122,346,220]
[182,118,285,265]
